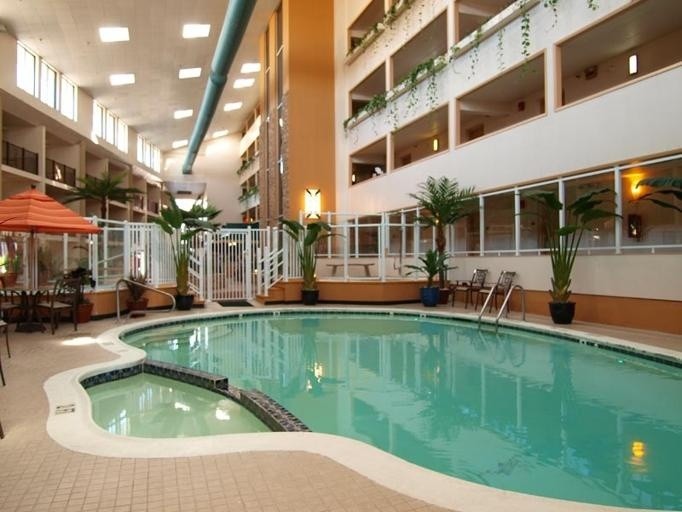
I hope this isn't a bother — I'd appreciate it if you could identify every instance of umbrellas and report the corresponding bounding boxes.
[0,184,106,291]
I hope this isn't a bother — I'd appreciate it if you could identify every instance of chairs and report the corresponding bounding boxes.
[0,284,78,334]
[451,269,515,313]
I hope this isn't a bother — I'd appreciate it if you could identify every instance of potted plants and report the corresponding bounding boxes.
[404,179,476,307]
[416,322,467,440]
[547,341,577,422]
[523,181,615,325]
[127,271,149,319]
[156,190,224,310]
[64,265,97,323]
[286,317,324,396]
[282,214,346,304]
